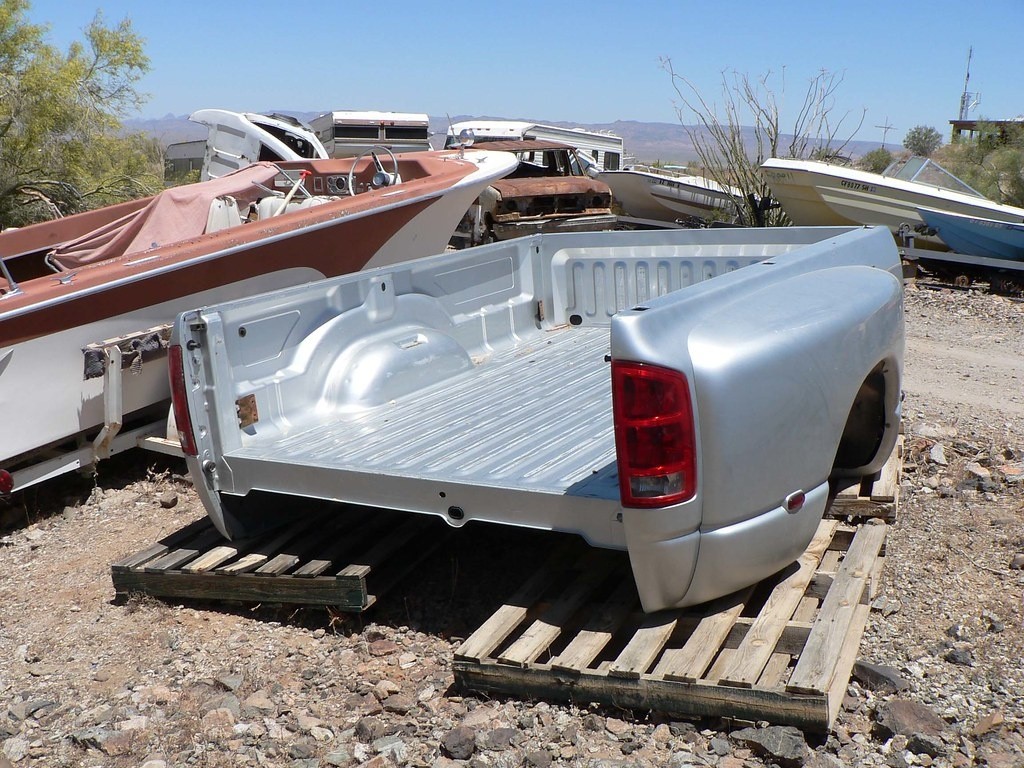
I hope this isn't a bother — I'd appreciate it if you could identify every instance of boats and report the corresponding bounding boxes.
[597,165,780,226]
[914,207,1024,279]
[759,155,984,253]
[0,148,522,487]
